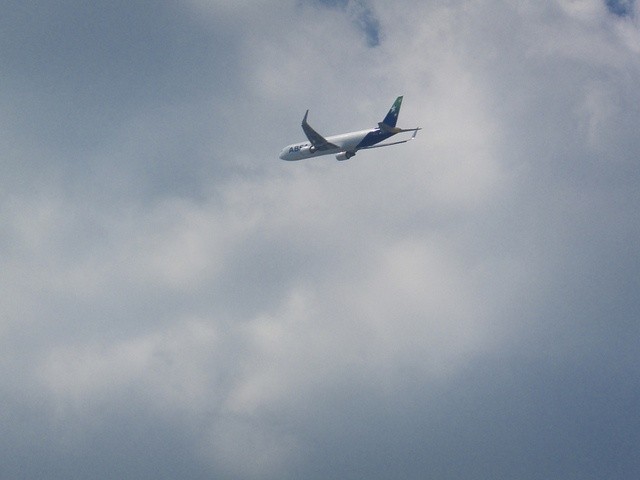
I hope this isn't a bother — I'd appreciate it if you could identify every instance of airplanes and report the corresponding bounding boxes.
[279,94,422,163]
